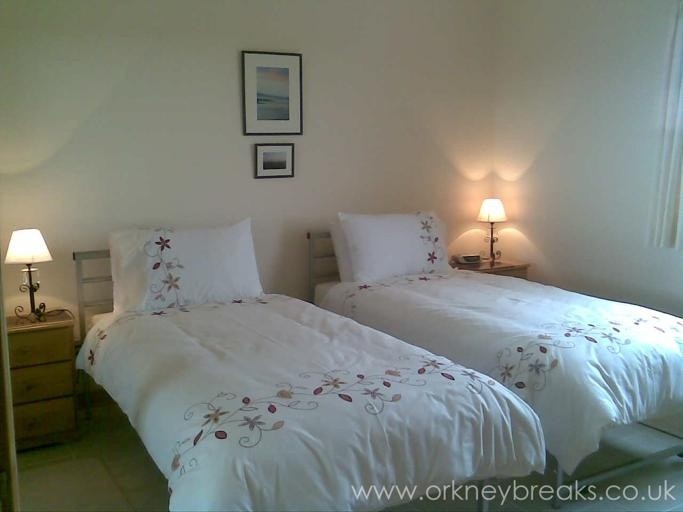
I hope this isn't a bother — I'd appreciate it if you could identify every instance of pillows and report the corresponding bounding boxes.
[109,216,266,313]
[328,212,457,283]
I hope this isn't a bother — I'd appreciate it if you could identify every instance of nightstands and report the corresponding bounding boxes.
[5,309,75,453]
[451,260,531,280]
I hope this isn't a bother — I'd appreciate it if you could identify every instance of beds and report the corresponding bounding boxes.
[72,249,547,511]
[306,230,683,508]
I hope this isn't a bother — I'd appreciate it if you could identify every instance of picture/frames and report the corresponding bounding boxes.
[255,142,294,179]
[241,51,303,136]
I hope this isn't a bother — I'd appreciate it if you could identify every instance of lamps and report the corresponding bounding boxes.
[477,198,509,262]
[3,227,55,321]
